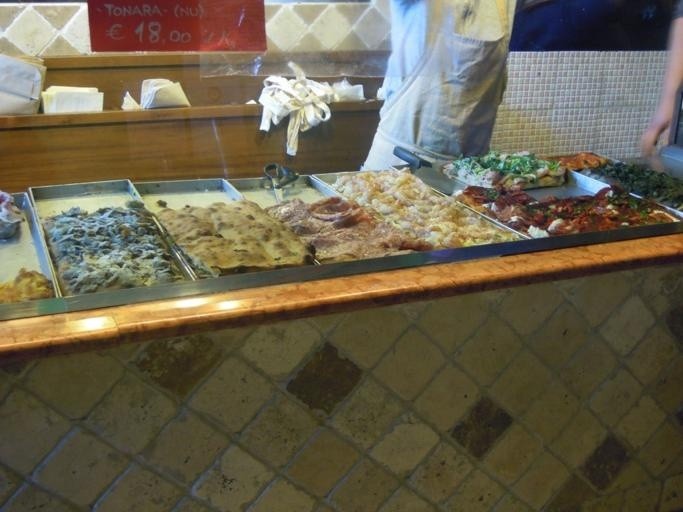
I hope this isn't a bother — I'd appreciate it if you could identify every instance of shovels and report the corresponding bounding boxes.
[393,146,468,195]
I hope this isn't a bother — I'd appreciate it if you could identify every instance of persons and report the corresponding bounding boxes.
[638,0,683,165]
[359,0,517,174]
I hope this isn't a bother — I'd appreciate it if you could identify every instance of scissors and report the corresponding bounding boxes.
[263,162,298,204]
